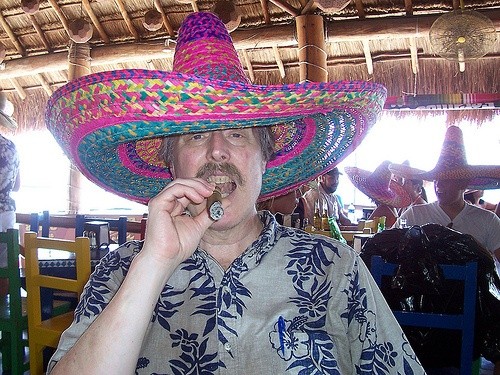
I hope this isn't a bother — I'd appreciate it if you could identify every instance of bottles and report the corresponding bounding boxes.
[348,202,355,223]
[373,216,408,233]
[303,209,329,230]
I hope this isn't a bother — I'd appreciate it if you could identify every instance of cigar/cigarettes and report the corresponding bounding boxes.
[207,186,224,221]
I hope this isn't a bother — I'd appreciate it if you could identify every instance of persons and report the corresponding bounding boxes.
[393,160,428,217]
[344,161,410,230]
[390,125,500,263]
[464,189,500,219]
[0,110,20,295]
[46,12,427,375]
[301,167,352,231]
[255,182,311,229]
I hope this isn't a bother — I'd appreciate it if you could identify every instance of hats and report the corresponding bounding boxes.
[389,125,500,189]
[46,12,388,205]
[0,92,19,131]
[327,167,343,175]
[344,159,412,207]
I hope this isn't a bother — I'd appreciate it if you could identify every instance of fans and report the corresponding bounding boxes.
[429,9,498,64]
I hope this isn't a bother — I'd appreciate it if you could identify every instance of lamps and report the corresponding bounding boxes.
[20,0,39,14]
[211,0,241,34]
[141,0,164,31]
[68,0,93,43]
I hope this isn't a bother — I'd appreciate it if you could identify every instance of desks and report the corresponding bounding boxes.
[20,243,121,372]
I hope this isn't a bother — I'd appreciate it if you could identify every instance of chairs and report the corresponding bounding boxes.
[0,208,147,375]
[306,217,479,375]
